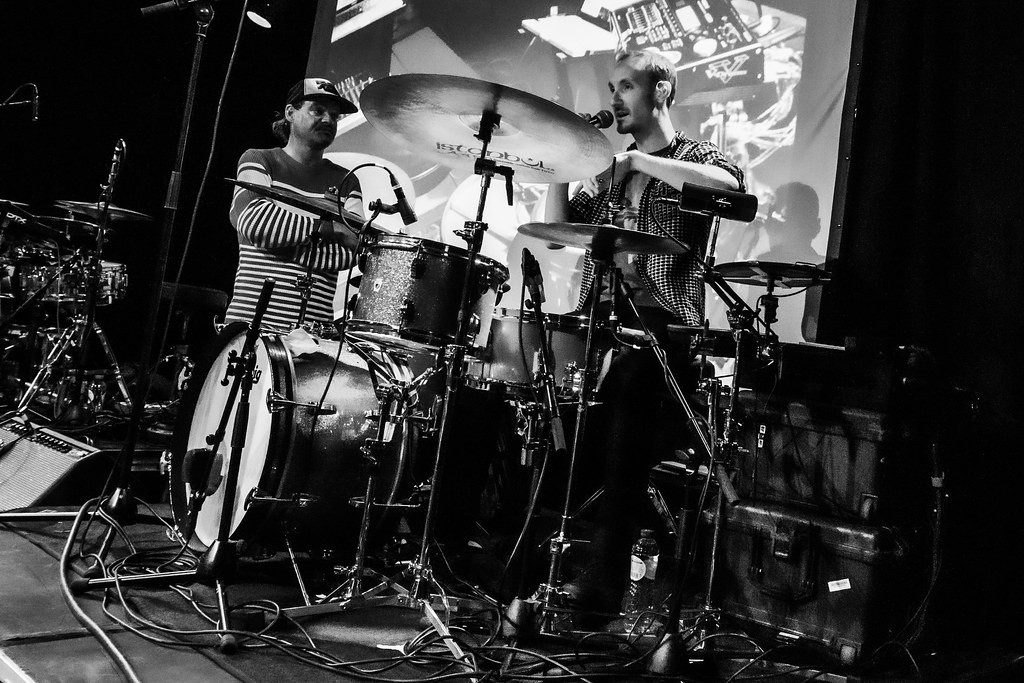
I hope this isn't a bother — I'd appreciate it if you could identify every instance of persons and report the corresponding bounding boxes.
[533,49,745,632]
[223,77,366,340]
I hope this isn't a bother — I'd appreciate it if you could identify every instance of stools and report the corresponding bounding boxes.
[107,282,228,409]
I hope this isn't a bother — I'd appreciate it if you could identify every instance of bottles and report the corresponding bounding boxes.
[87,374,106,413]
[624,528,660,618]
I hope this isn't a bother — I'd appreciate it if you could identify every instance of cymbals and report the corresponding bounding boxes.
[35,215,101,237]
[358,73,616,186]
[54,197,155,222]
[223,174,396,238]
[516,220,692,258]
[712,257,830,290]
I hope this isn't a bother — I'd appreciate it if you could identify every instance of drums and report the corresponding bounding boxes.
[485,304,654,409]
[342,236,513,363]
[28,261,131,304]
[166,319,424,569]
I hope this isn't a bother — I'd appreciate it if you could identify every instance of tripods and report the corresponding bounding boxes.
[17,122,787,683]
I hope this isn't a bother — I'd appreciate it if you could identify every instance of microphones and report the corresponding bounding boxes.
[588,110,614,129]
[389,174,418,226]
[521,249,546,304]
[32,93,42,120]
[181,447,211,540]
[108,142,122,183]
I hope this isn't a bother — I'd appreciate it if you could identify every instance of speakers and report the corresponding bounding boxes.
[0,410,120,512]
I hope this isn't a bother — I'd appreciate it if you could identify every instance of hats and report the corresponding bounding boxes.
[287,78,358,114]
